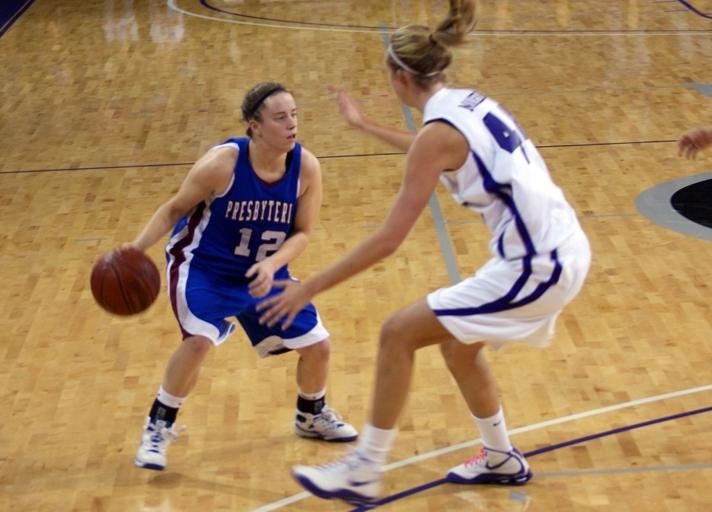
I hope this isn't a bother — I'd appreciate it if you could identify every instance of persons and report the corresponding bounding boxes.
[678,127,712,162]
[254,0,591,509]
[125,82,359,471]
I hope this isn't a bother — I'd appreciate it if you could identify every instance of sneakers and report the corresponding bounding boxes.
[134,416,176,470]
[295,405,358,443]
[291,452,385,508]
[445,448,533,484]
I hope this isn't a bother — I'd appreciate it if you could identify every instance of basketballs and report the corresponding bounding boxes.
[91,245,162,316]
